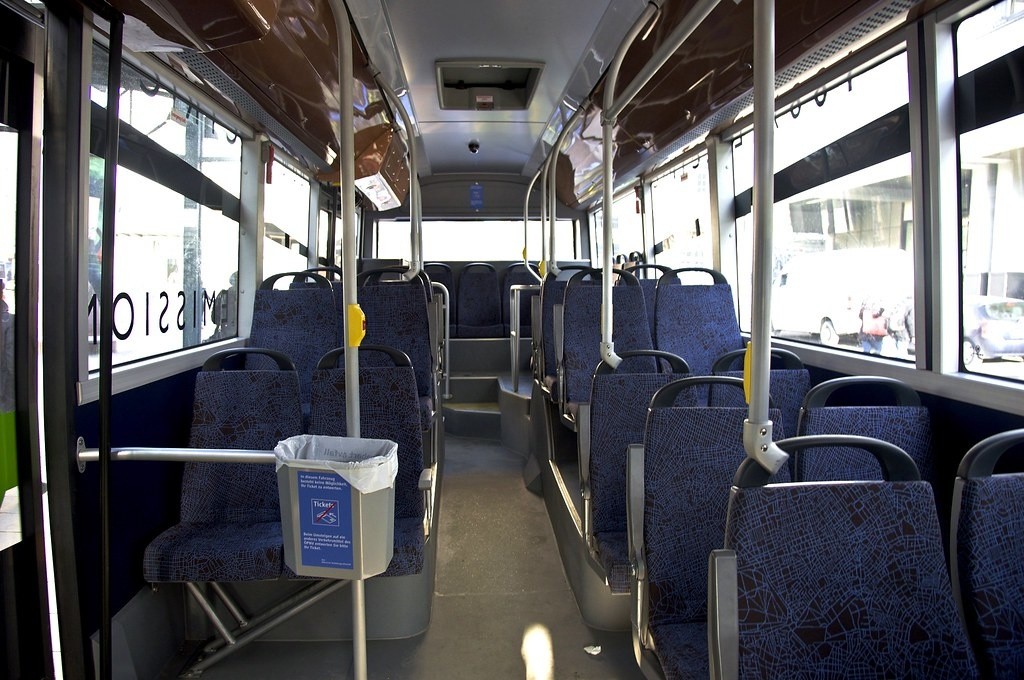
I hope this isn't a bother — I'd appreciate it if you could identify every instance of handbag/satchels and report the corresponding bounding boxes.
[862,309,888,336]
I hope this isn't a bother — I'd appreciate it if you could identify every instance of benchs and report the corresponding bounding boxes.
[140,263,455,678]
[459,261,1024,680]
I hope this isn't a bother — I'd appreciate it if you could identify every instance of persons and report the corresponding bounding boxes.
[859,291,887,355]
[0,277,15,506]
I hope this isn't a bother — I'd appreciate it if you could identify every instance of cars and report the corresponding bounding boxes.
[962,294,1024,368]
[771,246,914,347]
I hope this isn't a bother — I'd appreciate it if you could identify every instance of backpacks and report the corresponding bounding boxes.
[887,300,915,331]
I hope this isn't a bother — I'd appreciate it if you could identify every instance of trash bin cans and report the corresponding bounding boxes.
[274,435,398,581]
